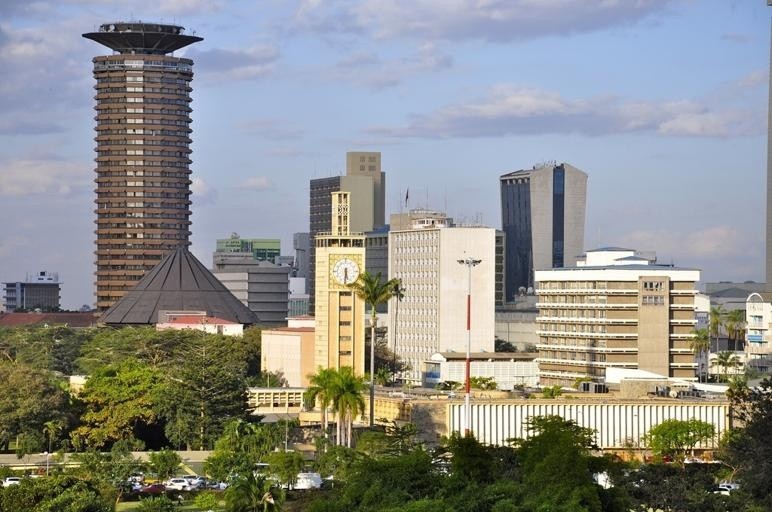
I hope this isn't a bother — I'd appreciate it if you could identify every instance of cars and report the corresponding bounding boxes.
[3,471,229,498]
[707,479,739,499]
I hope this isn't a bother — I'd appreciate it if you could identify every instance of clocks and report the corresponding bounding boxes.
[333,259,359,286]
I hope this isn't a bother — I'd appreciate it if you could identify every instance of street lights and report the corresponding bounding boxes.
[457,255,483,440]
